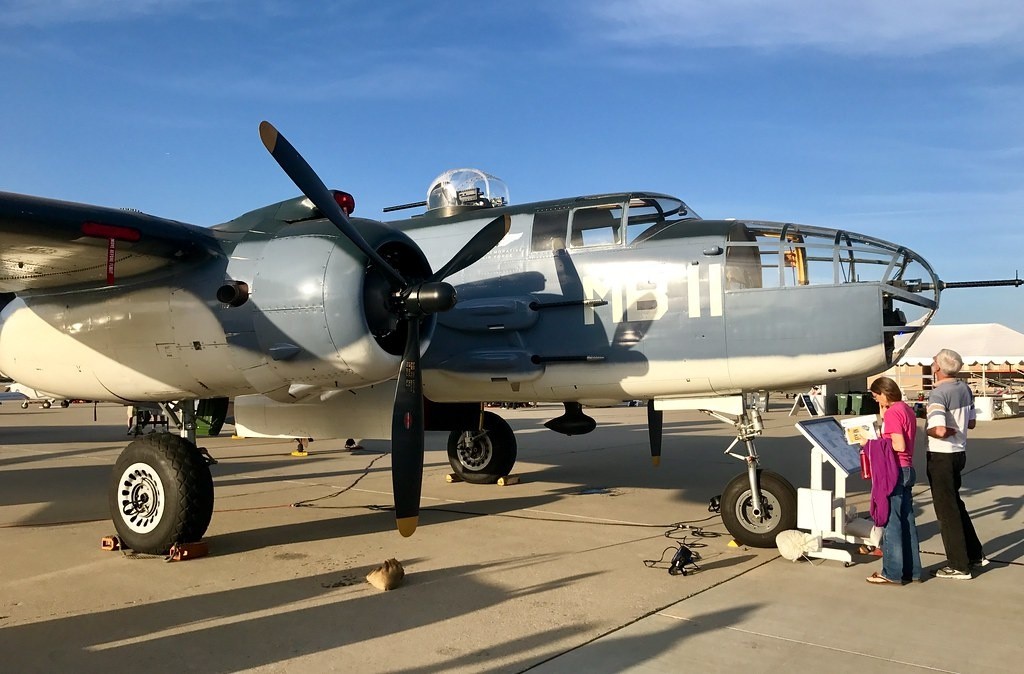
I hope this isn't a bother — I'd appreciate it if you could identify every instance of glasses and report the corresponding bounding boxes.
[873,394,878,400]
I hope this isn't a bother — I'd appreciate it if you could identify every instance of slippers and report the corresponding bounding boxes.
[903,579,923,584]
[866,572,902,586]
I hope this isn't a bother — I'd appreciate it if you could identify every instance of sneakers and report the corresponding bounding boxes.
[969,552,992,572]
[929,565,972,579]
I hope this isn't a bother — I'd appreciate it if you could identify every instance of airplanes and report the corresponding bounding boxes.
[1,121,1023,553]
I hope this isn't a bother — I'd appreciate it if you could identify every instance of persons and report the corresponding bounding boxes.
[925,349,990,579]
[860,377,923,587]
[127,402,166,435]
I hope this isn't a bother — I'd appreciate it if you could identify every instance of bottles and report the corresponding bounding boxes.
[860,450,871,480]
